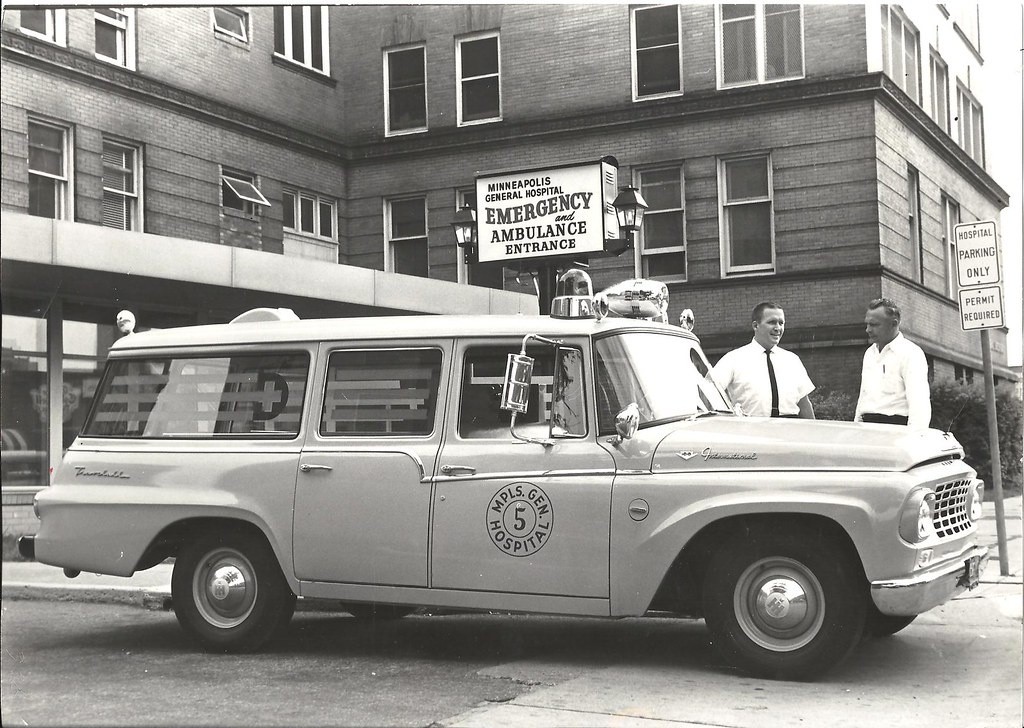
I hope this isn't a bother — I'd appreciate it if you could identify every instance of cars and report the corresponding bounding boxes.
[20,270,991,683]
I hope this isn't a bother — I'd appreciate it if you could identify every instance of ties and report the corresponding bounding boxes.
[764,351,779,417]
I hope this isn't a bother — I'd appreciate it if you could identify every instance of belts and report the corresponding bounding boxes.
[861,413,908,424]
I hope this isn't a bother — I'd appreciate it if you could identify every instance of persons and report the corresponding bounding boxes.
[854,297,932,428]
[712,298,818,421]
